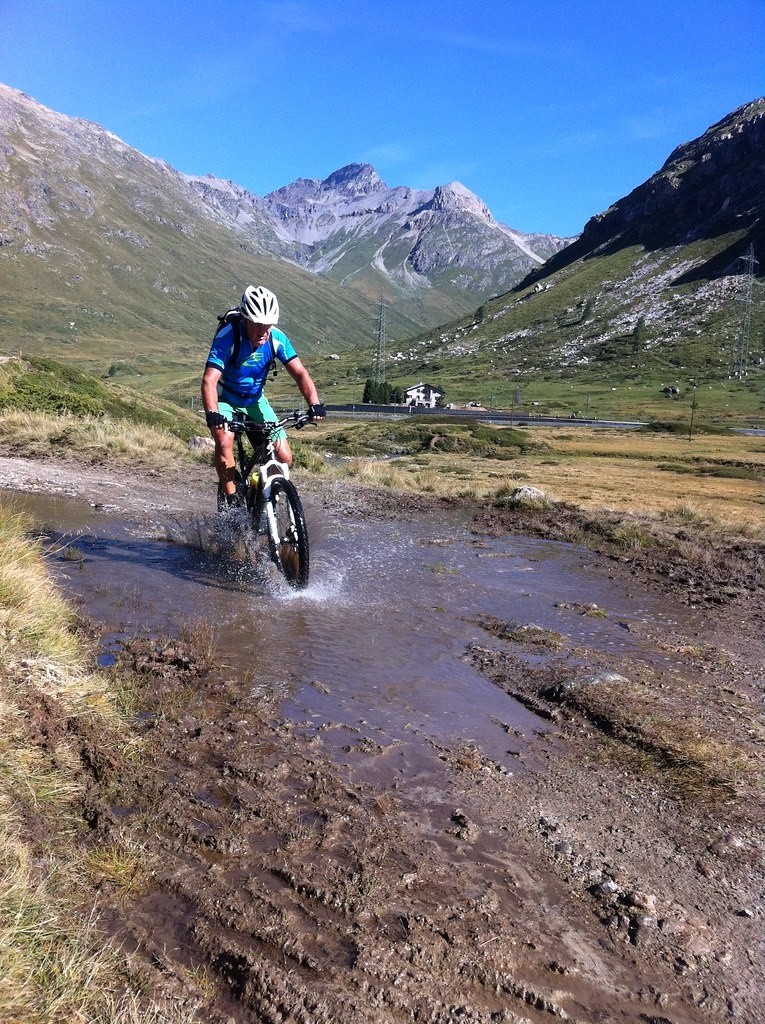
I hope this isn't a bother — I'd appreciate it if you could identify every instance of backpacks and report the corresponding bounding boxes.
[212,307,277,372]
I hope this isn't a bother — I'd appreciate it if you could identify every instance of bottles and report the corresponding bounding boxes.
[247,472,259,507]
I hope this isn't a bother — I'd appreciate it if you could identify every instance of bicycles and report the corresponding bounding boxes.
[208,410,310,591]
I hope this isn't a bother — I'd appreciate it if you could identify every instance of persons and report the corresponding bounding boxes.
[204,284,326,534]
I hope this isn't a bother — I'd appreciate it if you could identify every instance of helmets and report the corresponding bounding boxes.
[240,285,280,325]
